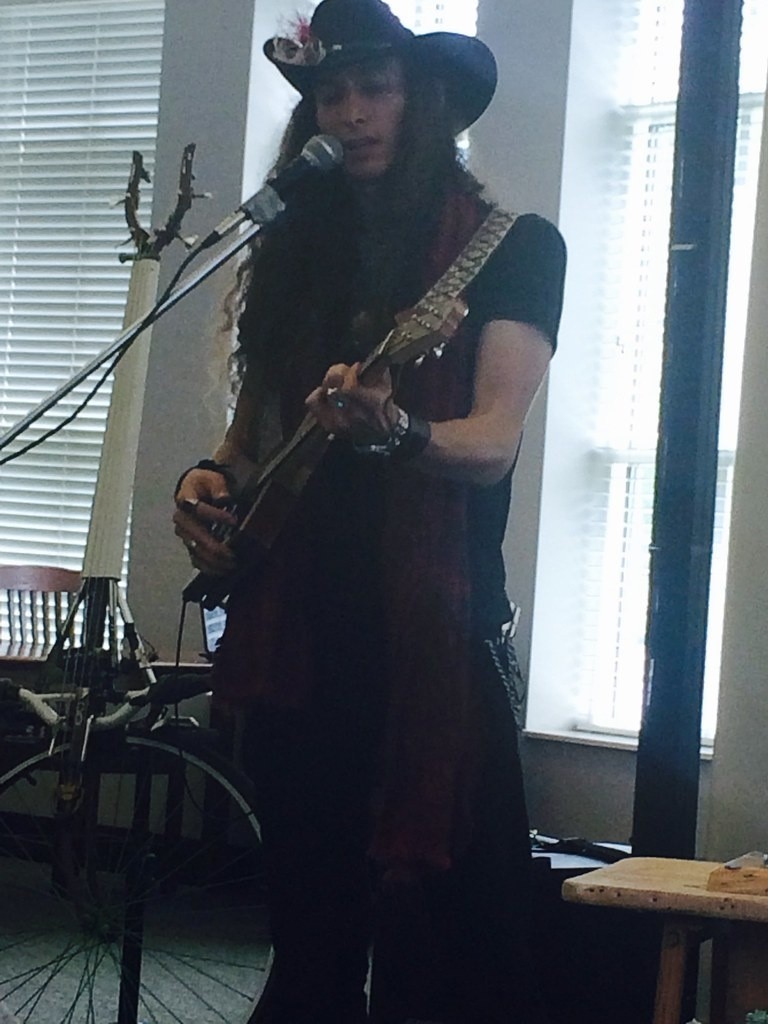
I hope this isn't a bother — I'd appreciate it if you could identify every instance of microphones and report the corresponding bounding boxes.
[201,134,346,248]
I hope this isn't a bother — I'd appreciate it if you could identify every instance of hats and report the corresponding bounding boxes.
[262,0,498,138]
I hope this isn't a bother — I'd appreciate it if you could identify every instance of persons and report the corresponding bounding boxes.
[171,0,572,1024]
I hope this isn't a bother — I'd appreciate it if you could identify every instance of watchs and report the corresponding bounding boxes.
[368,407,410,463]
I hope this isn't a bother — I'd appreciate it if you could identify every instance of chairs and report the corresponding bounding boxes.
[0,565,188,898]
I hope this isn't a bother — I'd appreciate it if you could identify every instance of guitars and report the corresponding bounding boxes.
[181,293,470,613]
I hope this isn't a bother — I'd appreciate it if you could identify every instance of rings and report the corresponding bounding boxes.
[182,497,198,517]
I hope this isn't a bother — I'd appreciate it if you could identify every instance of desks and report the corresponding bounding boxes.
[562,857,768,1024]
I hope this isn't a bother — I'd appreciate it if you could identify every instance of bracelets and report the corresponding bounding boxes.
[392,413,430,461]
[174,458,236,500]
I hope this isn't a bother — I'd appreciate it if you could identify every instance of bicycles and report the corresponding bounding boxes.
[1,671,291,1024]
